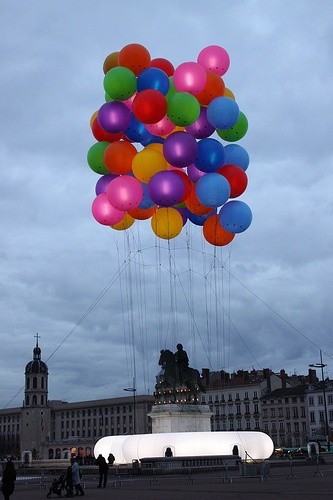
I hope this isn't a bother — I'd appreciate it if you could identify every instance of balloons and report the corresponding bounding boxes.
[86,42,254,247]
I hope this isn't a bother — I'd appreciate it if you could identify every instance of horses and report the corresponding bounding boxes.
[158,348,207,403]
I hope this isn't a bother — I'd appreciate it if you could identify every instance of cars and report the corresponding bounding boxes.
[279,448,305,459]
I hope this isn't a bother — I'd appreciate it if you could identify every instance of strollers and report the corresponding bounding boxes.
[46,470,69,498]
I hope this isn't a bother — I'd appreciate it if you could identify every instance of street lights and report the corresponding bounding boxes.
[309,350,330,451]
[123,377,136,435]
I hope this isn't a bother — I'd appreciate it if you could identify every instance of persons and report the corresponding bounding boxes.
[0,462,16,500]
[65,467,73,497]
[95,454,109,489]
[70,459,84,497]
[173,343,189,368]
[70,452,95,466]
